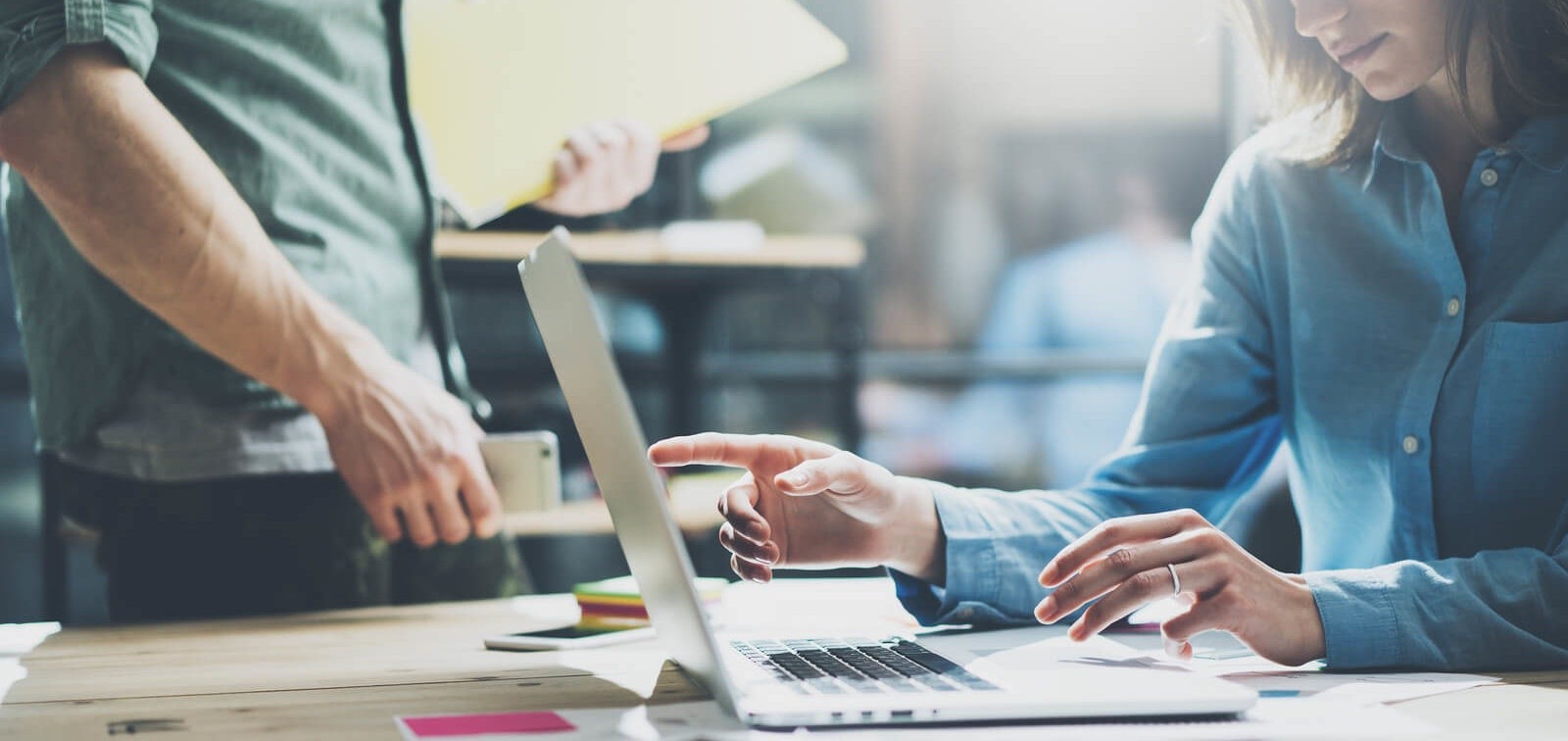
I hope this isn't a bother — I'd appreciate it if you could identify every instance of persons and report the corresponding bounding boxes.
[0,0,711,630]
[647,0,1567,674]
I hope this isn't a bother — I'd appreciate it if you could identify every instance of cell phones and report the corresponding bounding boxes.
[480,430,562,514]
[485,621,646,652]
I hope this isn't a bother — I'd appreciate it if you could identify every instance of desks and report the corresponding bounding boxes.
[429,229,891,454]
[0,580,1568,741]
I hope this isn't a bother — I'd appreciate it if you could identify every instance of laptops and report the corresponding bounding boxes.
[517,226,1259,729]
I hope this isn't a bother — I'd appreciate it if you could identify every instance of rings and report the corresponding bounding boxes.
[1167,563,1183,598]
